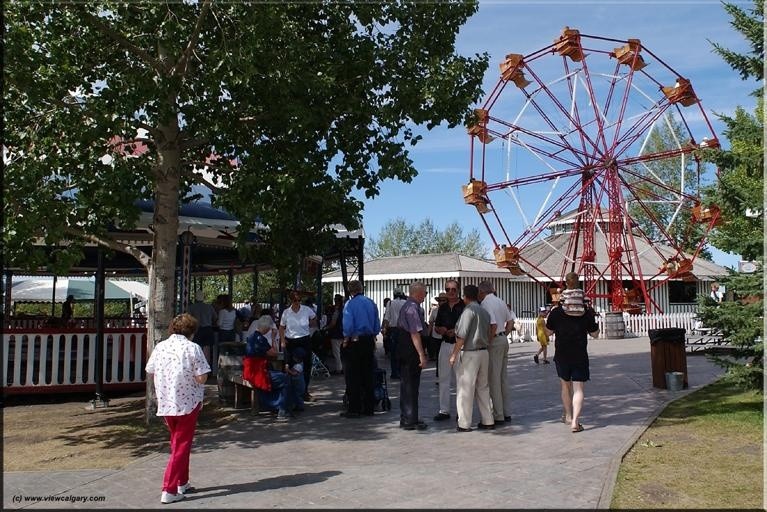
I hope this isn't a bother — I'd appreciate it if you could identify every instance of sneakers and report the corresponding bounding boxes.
[161,483,190,504]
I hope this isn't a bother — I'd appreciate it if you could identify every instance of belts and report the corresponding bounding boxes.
[479,347,488,350]
[495,332,505,336]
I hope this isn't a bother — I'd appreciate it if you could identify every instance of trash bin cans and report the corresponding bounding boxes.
[217,342,245,405]
[649,328,688,388]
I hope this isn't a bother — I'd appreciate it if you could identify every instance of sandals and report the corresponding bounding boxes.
[534,355,550,364]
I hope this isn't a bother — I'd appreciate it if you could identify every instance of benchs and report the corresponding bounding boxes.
[224,365,260,413]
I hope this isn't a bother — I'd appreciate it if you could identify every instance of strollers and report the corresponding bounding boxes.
[343,356,390,413]
[310,334,332,377]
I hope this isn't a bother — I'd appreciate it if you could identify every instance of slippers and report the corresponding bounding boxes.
[560,416,584,432]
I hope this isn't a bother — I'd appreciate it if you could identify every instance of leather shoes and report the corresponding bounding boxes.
[402,422,426,430]
[434,413,511,432]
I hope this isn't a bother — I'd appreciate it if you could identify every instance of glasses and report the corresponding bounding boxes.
[445,288,456,292]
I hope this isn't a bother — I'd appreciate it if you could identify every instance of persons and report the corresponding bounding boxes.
[497,244,506,257]
[466,178,476,190]
[674,78,680,89]
[144,312,212,505]
[505,54,525,80]
[700,136,710,148]
[709,282,723,310]
[61,295,75,319]
[668,258,681,274]
[533,272,600,432]
[186,279,519,435]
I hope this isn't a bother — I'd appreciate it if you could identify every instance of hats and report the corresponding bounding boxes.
[394,288,403,295]
